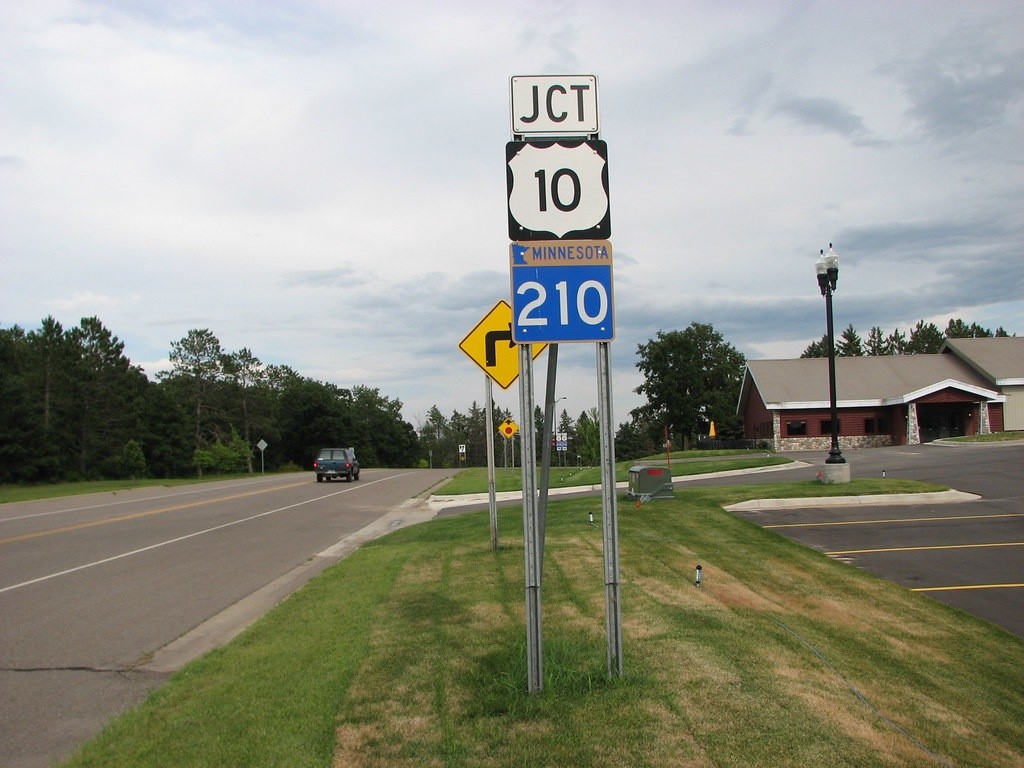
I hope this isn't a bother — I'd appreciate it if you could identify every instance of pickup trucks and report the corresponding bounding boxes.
[314,447,360,482]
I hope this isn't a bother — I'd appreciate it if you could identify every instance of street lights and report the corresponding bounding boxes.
[815,242,852,484]
[554,396,568,457]
